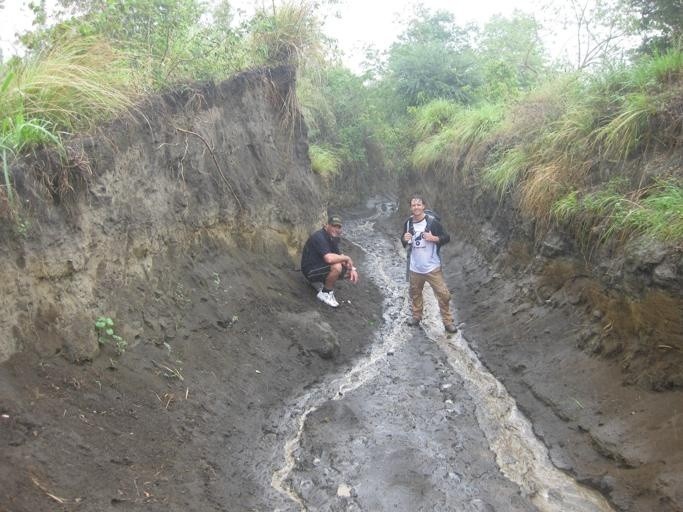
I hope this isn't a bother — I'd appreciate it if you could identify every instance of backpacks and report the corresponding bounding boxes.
[407,210,440,232]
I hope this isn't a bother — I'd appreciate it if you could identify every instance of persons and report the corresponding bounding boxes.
[298,213,360,309]
[398,193,460,334]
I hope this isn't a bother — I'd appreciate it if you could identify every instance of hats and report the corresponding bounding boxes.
[328,215,344,226]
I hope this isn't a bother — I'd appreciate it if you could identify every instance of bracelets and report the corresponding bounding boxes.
[348,267,357,272]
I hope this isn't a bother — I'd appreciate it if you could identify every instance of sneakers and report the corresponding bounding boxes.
[406,318,418,326]
[445,324,457,333]
[310,281,339,307]
